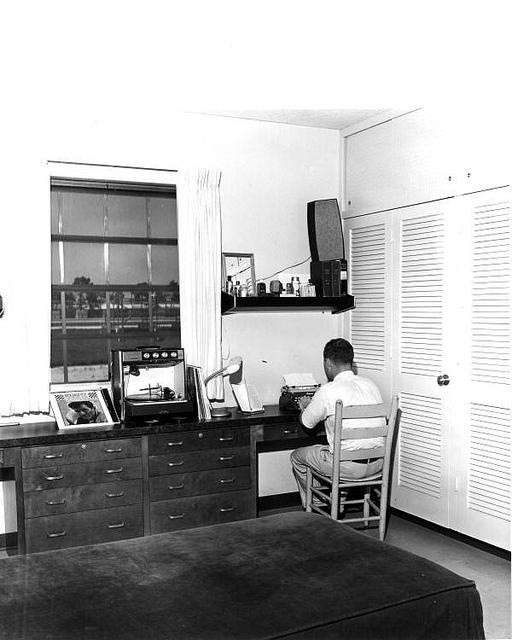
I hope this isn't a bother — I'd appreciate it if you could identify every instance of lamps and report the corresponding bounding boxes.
[204,356,243,417]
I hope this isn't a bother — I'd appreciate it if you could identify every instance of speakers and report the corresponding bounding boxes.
[307,199,345,262]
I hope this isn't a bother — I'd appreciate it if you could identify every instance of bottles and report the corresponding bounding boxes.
[286,277,316,297]
[226,275,251,297]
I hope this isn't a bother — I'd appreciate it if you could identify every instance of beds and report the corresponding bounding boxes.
[0,510,485,640]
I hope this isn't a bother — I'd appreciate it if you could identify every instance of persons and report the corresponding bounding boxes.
[289,338,389,521]
[64,400,109,427]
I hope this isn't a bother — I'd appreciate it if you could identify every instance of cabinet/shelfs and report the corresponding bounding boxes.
[338,106,512,558]
[0,403,327,555]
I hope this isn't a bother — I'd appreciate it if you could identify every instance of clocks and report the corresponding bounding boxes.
[221,252,257,298]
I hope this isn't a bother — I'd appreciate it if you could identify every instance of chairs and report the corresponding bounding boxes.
[306,396,398,541]
